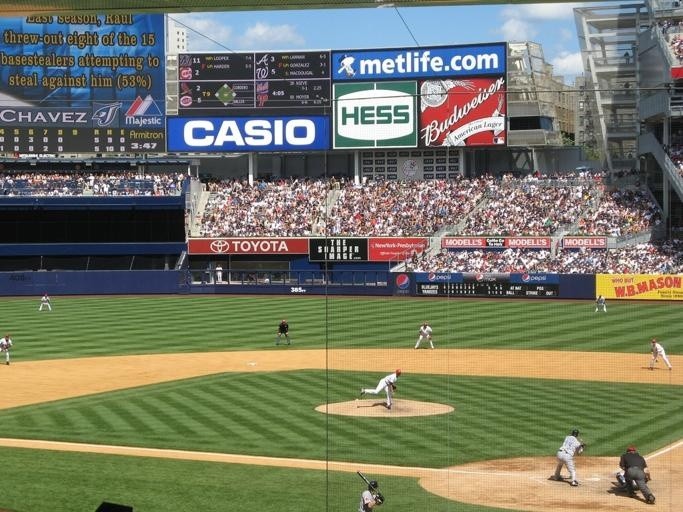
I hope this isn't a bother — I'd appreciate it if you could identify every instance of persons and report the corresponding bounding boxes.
[214,263,223,283]
[414,322,434,349]
[662,142,682,165]
[275,318,291,345]
[0,170,197,198]
[38,293,51,312]
[359,370,401,410]
[358,481,382,512]
[619,447,654,504]
[201,165,682,273]
[593,294,605,312]
[0,332,12,363]
[649,339,671,370]
[552,429,585,486]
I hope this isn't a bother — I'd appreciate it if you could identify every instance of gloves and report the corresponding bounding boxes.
[376,494,384,501]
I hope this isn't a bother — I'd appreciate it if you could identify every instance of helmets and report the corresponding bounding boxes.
[368,482,376,489]
[627,447,636,453]
[572,429,579,435]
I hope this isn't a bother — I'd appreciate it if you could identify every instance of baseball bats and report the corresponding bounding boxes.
[357,471,380,496]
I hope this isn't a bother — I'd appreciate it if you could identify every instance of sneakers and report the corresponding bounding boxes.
[571,481,578,485]
[360,388,365,395]
[648,496,655,504]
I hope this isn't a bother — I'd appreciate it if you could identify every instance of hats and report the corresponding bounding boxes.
[396,370,400,376]
